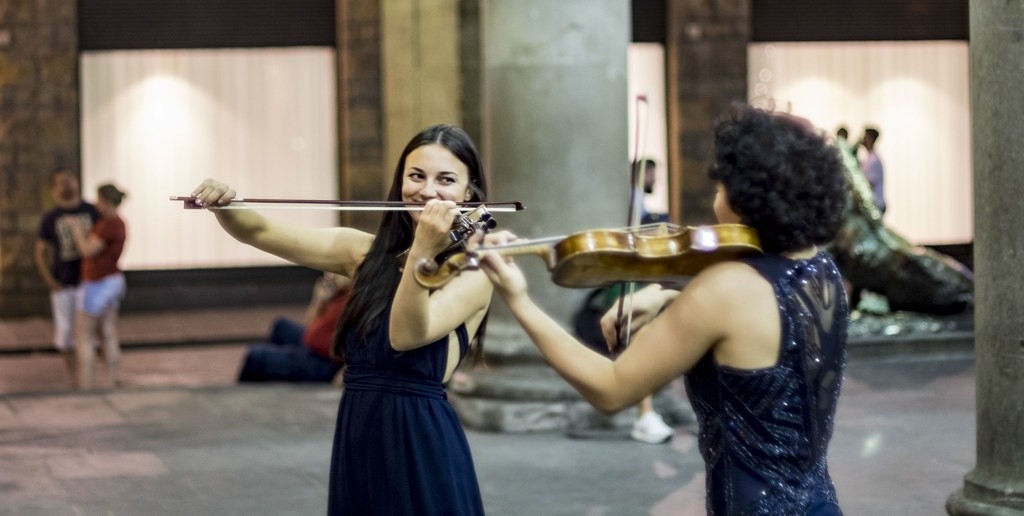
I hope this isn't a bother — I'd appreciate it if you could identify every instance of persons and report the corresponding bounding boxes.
[836,128,886,321]
[191,123,495,516]
[34,171,126,392]
[464,97,852,515]
[234,272,354,389]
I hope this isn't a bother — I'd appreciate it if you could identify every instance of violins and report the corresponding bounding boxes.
[412,217,763,289]
[394,202,489,273]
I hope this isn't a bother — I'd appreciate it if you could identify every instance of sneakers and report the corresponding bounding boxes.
[629,411,676,445]
